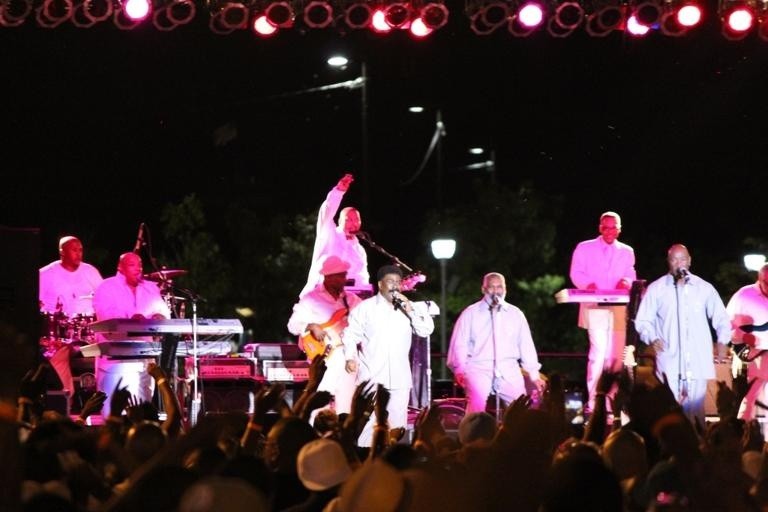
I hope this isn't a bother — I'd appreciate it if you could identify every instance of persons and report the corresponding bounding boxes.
[634,244,730,443]
[569,212,638,426]
[38,236,104,415]
[343,265,435,446]
[447,272,546,417]
[726,265,768,438]
[292,173,375,301]
[91,253,170,418]
[288,255,363,363]
[1,333,768,512]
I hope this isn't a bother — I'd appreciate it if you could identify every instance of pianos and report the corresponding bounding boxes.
[81,341,238,357]
[88,318,244,335]
[555,287,647,304]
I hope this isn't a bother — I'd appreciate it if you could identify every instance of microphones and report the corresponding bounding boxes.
[136,273,161,282]
[133,223,144,255]
[393,291,407,308]
[355,230,366,239]
[492,293,502,305]
[679,267,690,282]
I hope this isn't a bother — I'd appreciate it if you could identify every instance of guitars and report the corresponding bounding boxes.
[299,270,426,363]
[733,321,768,362]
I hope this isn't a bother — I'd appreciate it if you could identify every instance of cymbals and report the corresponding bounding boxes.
[143,270,188,281]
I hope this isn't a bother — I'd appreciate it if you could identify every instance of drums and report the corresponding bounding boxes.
[39,311,95,340]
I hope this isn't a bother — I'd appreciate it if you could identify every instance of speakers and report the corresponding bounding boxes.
[190,378,262,414]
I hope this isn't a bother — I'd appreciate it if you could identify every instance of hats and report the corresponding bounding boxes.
[319,255,352,276]
[296,439,352,492]
